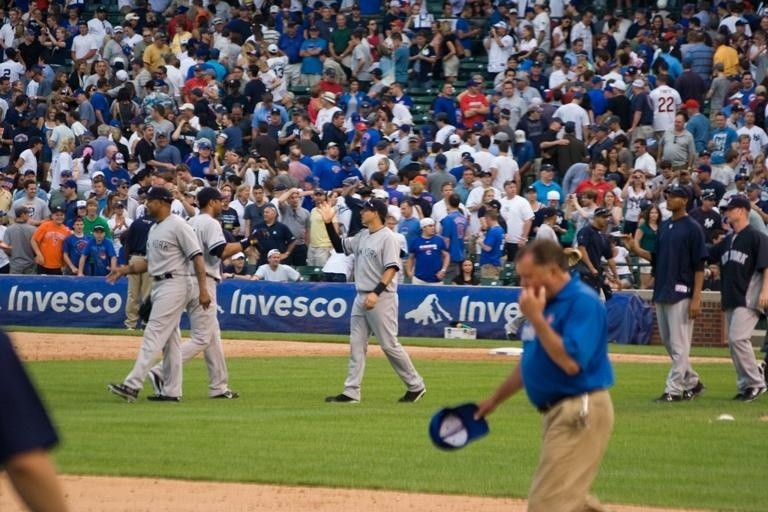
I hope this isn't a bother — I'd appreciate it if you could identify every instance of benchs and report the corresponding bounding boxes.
[273,31,520,129]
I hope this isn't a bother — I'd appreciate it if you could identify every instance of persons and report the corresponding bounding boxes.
[101,187,262,404]
[0,1,768,299]
[313,194,427,404]
[646,178,706,405]
[474,234,616,511]
[0,325,65,511]
[704,195,767,403]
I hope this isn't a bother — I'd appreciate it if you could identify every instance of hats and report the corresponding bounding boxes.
[429,403,489,452]
[12,2,766,262]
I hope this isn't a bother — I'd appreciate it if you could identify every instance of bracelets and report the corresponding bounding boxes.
[373,282,386,297]
[239,237,251,251]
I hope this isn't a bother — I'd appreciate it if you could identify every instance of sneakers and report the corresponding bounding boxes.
[653,391,684,403]
[741,382,766,402]
[326,394,359,403]
[734,391,741,400]
[109,369,180,403]
[686,380,704,402]
[214,391,238,399]
[397,389,426,403]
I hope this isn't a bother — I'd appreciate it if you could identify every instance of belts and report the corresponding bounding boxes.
[538,396,563,413]
[152,271,177,281]
